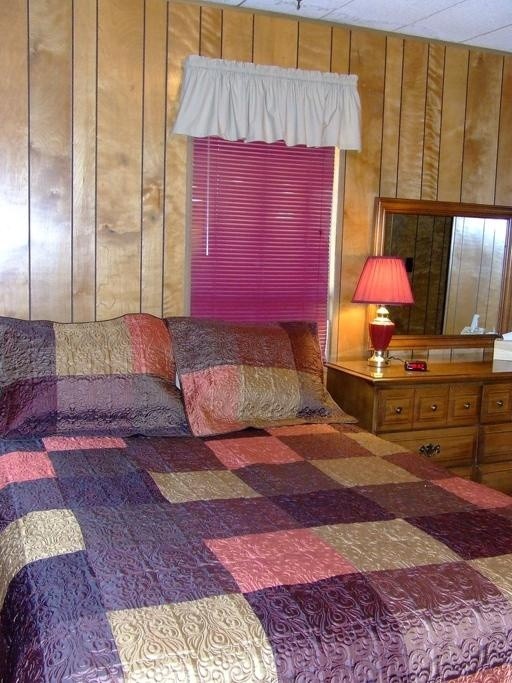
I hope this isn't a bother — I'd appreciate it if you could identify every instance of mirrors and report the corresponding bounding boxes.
[364,197,512,351]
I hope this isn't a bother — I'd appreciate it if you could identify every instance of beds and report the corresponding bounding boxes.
[0,424,512,683]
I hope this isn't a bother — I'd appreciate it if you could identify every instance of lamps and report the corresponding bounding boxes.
[351,257,415,367]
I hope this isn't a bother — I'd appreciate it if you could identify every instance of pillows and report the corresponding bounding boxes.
[163,318,359,438]
[0,313,194,439]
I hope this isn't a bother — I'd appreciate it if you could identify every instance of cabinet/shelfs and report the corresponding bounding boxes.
[325,361,512,497]
